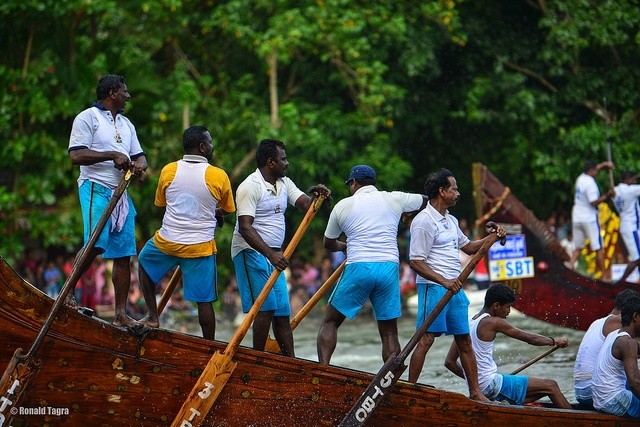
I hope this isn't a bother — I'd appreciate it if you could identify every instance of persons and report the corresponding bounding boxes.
[409,167,507,402]
[574,289,640,407]
[231,138,331,358]
[317,164,429,367]
[444,283,574,408]
[61,75,149,326]
[564,158,615,280]
[137,126,236,342]
[592,294,640,425]
[611,170,640,284]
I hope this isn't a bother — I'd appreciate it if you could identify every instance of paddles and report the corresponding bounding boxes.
[266,258,347,355]
[170,187,334,427]
[338,222,506,427]
[0,159,147,427]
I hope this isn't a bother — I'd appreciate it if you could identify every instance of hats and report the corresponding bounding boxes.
[344,164,376,183]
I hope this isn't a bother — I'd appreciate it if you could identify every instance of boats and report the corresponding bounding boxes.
[474,164,640,331]
[0,259,640,427]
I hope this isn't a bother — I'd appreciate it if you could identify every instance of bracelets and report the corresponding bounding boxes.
[550,335,556,347]
